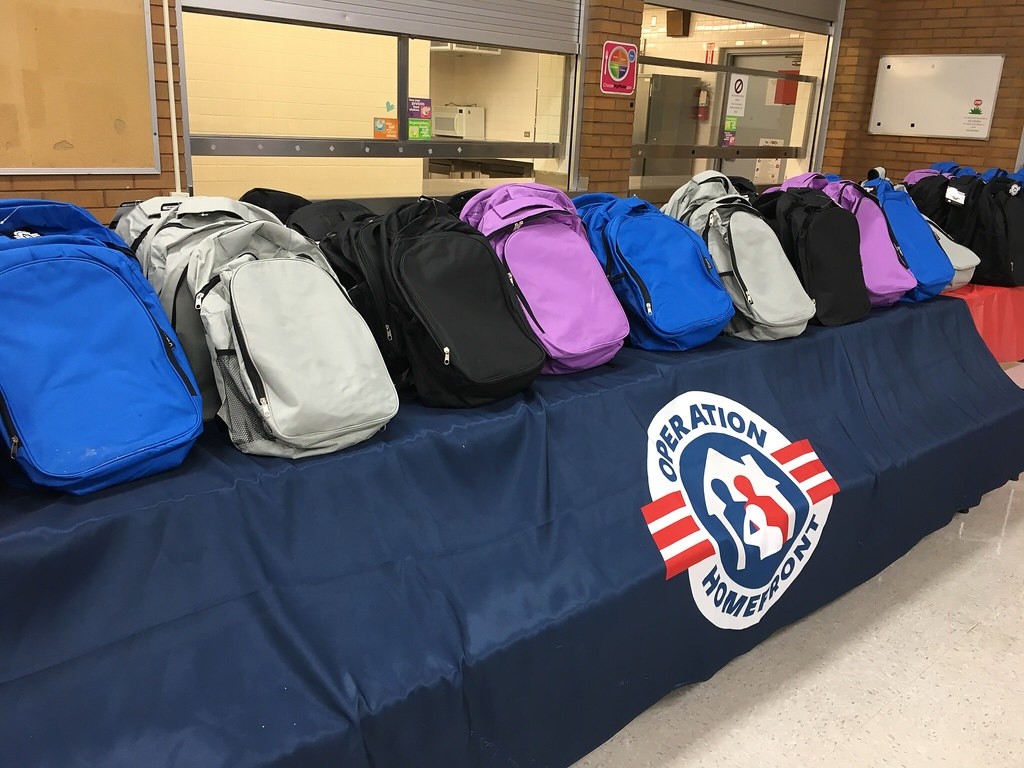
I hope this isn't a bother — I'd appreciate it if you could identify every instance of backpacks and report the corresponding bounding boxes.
[0,161,1024,498]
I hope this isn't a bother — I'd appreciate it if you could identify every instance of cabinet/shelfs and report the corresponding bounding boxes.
[0,283,1024,768]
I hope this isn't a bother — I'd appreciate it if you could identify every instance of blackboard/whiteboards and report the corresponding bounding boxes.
[866,54,1005,142]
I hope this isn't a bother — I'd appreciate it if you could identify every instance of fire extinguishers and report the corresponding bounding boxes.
[696,83,712,120]
